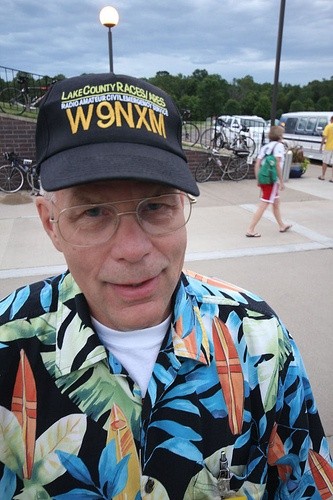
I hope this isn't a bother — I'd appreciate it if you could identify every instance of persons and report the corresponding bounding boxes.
[318,116,333,183]
[245,126,293,237]
[0,73,333,500]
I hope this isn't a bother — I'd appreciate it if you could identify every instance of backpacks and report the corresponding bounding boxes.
[257,142,278,185]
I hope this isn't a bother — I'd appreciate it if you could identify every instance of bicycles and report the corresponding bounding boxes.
[181,109,200,147]
[0,151,43,196]
[198,118,257,159]
[194,146,250,183]
[0,76,57,117]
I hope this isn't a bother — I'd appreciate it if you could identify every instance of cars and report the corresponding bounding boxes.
[212,115,280,155]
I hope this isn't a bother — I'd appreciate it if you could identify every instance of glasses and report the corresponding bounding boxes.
[48,193,197,248]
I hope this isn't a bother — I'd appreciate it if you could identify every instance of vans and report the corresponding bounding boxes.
[279,112,333,167]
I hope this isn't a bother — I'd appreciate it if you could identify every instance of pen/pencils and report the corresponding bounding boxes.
[219,451,230,480]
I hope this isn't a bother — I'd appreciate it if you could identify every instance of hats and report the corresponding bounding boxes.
[31,73,202,197]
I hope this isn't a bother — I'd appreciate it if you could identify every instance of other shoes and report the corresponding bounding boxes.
[329,180,333,183]
[279,224,292,233]
[245,232,261,237]
[318,176,325,181]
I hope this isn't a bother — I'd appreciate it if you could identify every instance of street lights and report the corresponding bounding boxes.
[99,5,119,75]
